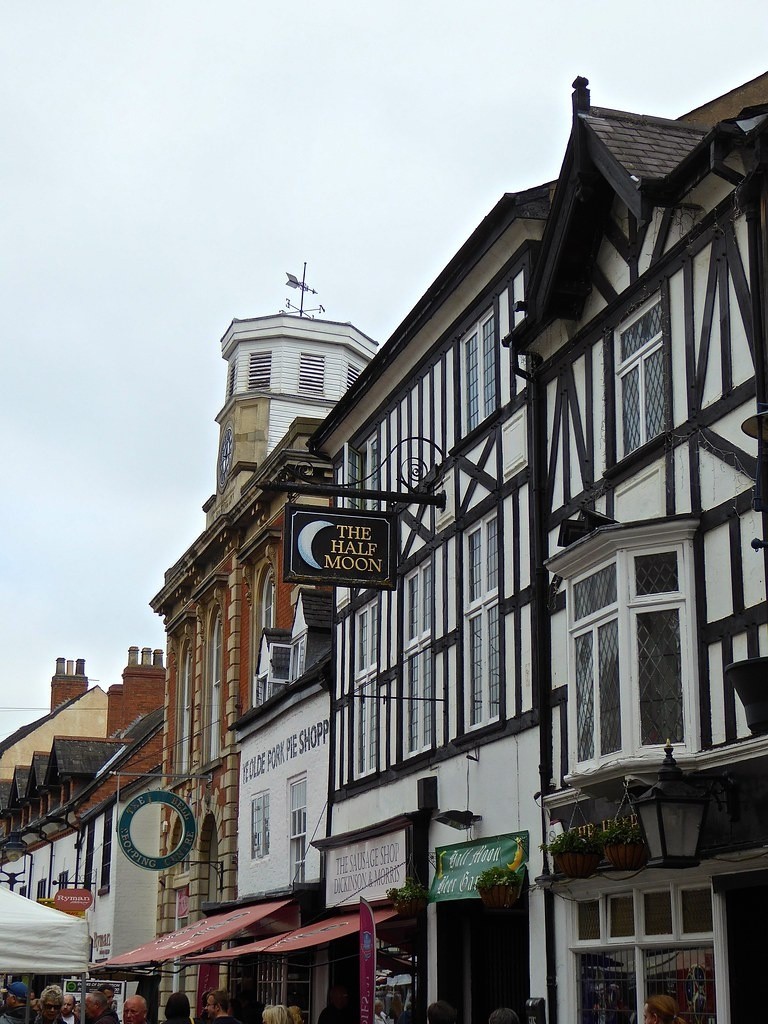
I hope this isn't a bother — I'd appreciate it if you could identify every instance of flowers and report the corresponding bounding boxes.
[593,819,646,851]
[473,865,523,891]
[538,822,605,858]
[386,877,440,909]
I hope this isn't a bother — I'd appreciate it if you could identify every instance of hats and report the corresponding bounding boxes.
[5,982,28,998]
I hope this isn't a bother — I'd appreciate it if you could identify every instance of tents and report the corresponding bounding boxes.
[0,883,89,1024]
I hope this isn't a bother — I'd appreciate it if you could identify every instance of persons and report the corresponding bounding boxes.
[317,986,360,1024]
[489,1008,521,1024]
[374,995,418,1024]
[0,981,305,1024]
[643,995,686,1024]
[426,1001,457,1024]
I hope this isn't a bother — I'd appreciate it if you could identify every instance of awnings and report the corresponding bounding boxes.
[103,897,296,973]
[172,904,411,968]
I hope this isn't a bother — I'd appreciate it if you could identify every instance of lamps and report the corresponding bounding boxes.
[632,737,740,869]
[432,809,482,831]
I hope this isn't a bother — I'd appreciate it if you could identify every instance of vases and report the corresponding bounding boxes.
[606,843,648,871]
[560,850,602,878]
[478,885,519,908]
[396,898,429,919]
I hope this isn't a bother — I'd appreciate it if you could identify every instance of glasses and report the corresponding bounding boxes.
[206,1003,222,1007]
[43,1003,62,1010]
